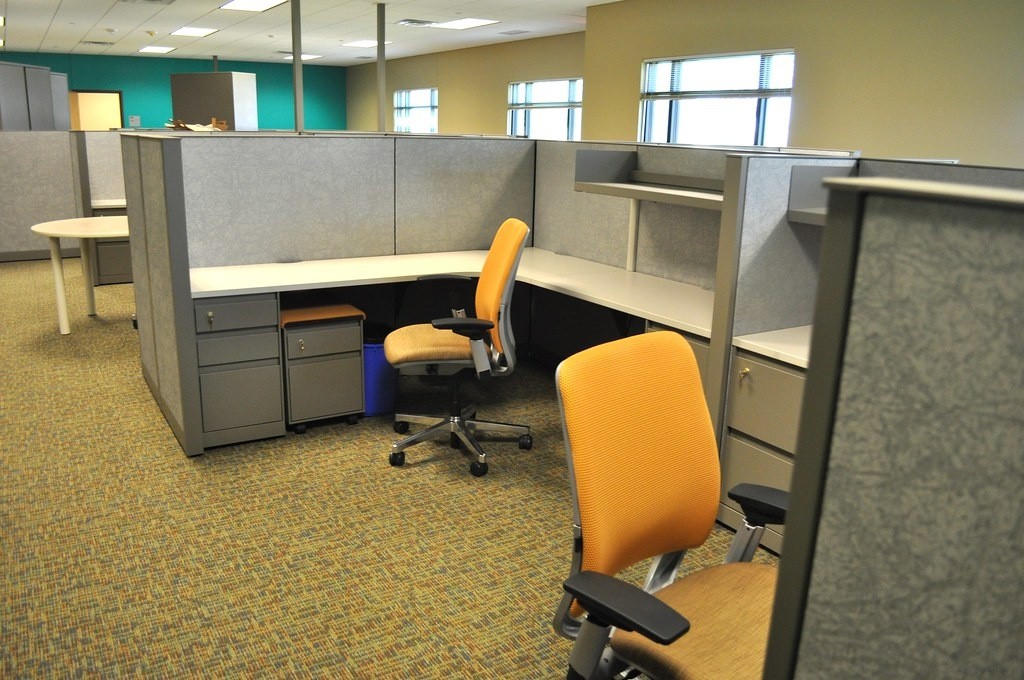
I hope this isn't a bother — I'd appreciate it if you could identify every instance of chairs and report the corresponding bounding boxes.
[552,331,791,680]
[388,219,533,477]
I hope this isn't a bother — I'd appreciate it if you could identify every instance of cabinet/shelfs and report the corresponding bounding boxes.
[170,72,261,132]
[0,61,72,131]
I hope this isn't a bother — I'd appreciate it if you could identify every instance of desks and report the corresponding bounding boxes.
[69,129,1022,680]
[31,216,129,334]
